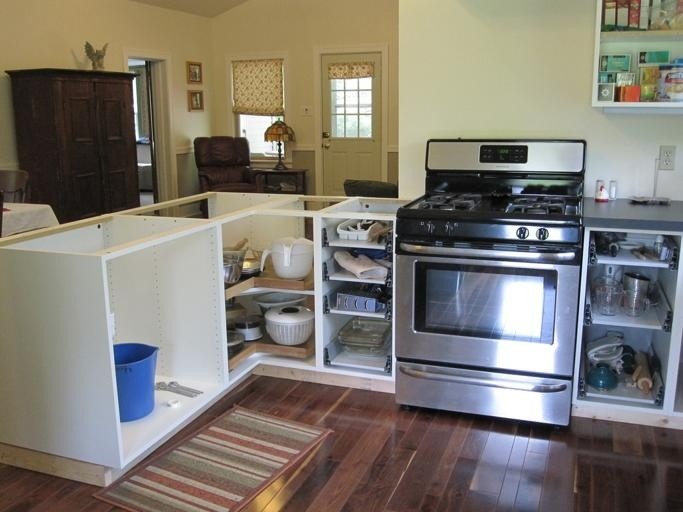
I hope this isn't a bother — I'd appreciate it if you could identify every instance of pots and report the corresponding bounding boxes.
[263,305,315,347]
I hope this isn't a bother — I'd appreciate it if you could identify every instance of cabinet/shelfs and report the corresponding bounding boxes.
[0,190,411,489]
[568,192,683,439]
[588,0,682,110]
[3,64,142,225]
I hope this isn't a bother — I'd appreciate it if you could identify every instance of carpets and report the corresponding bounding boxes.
[90,401,336,512]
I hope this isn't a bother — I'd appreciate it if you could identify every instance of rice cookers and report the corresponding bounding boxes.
[258,237,313,279]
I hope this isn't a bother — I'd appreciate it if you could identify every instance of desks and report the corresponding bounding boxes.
[250,166,310,196]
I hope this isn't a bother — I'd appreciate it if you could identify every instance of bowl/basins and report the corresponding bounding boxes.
[222,246,247,261]
[221,254,242,284]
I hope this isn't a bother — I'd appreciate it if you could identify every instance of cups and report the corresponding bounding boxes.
[590,263,652,320]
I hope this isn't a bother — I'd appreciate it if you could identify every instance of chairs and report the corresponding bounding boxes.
[0,168,30,203]
[189,133,267,225]
[341,176,397,200]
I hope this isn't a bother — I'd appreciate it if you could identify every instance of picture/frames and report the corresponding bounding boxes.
[185,60,202,86]
[187,89,204,114]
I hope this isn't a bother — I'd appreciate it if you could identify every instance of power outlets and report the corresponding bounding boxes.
[656,144,675,172]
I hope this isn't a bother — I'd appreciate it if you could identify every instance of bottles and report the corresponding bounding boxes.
[594,180,617,203]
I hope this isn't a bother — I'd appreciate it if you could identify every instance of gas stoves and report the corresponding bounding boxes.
[393,194,584,246]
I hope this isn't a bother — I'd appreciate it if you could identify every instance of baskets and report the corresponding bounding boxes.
[263,316,315,347]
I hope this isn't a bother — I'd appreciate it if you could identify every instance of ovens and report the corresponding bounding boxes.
[389,240,578,430]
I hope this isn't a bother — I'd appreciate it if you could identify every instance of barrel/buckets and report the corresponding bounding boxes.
[113,343,160,422]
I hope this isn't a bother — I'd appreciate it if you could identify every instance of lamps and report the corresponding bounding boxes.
[261,117,298,171]
